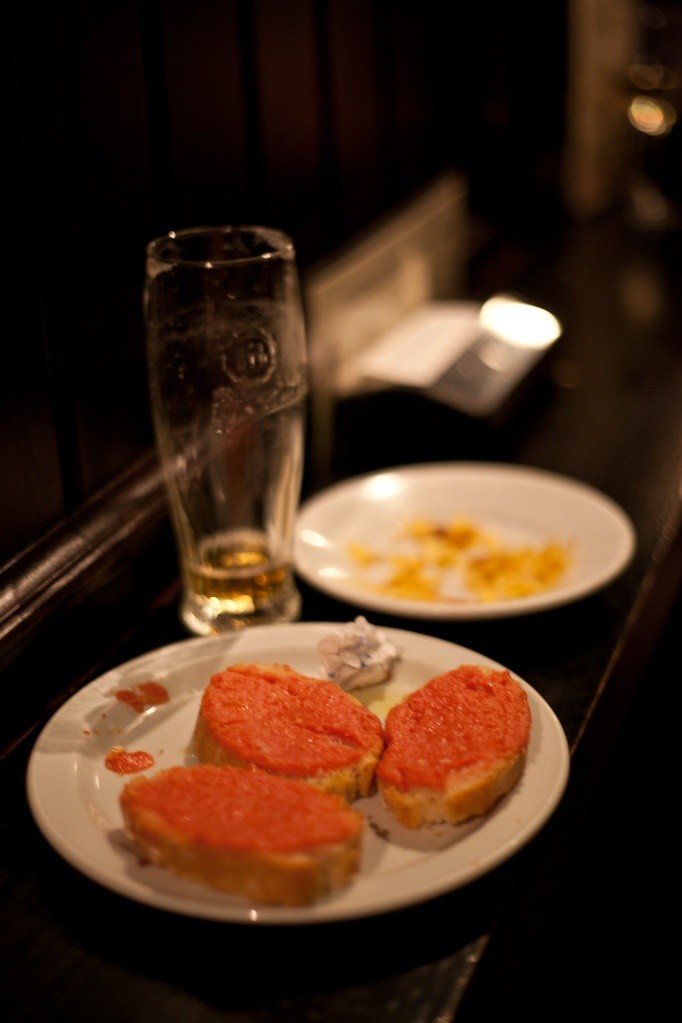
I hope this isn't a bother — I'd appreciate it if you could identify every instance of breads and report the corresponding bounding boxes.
[374,664,531,828]
[119,764,365,907]
[192,662,385,803]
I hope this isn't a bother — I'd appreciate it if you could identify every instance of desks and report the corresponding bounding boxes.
[0,244,682,1023]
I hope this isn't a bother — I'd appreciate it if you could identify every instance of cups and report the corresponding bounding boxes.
[145,228,304,636]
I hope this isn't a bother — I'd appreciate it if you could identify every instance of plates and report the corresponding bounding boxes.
[28,623,570,922]
[295,461,635,617]
